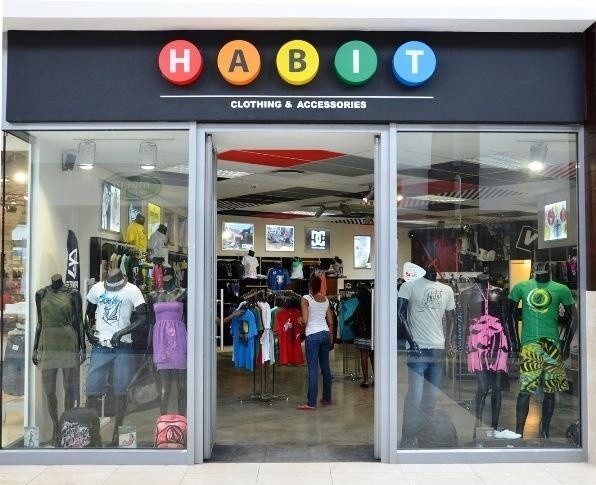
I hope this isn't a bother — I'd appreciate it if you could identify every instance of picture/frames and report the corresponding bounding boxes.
[353,234,371,269]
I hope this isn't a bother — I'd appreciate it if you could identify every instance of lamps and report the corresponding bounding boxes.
[517,139,576,171]
[72,135,174,170]
[363,184,403,204]
[5,205,17,213]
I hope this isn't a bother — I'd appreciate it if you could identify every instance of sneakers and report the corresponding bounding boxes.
[493,429,522,440]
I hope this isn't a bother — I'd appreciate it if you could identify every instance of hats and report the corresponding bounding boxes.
[104,268,127,291]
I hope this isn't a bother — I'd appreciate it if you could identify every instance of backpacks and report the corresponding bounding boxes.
[420,410,458,448]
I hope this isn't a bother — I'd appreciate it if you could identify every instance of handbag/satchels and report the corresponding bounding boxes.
[152,415,187,449]
[54,407,102,448]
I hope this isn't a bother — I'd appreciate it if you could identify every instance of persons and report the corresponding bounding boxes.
[509,270,579,438]
[84,268,147,446]
[461,273,514,444]
[217,307,245,327]
[397,267,457,449]
[345,287,375,387]
[145,275,187,416]
[297,273,333,409]
[32,274,87,445]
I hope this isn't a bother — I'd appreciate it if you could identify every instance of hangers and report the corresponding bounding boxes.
[239,289,301,313]
[113,241,134,254]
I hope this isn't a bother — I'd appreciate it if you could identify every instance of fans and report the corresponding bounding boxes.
[300,201,351,218]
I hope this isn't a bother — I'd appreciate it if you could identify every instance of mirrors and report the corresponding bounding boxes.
[0,131,36,448]
